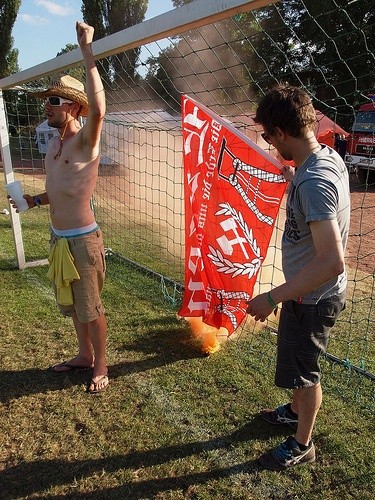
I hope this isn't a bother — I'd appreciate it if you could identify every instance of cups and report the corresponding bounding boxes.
[5,181,30,212]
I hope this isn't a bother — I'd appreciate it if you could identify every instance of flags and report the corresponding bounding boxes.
[177,96,288,338]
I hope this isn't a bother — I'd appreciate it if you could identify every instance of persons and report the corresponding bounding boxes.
[245,84,350,473]
[7,20,109,393]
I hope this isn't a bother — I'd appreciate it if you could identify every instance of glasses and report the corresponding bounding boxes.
[260,126,278,144]
[44,96,75,106]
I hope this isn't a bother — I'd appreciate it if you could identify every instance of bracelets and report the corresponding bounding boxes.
[33,196,41,207]
[266,292,279,317]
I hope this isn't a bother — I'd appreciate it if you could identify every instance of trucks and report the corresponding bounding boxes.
[344,102,375,183]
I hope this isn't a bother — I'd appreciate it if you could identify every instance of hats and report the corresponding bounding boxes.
[30,74,89,117]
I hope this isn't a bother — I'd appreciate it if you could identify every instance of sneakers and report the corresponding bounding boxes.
[261,403,299,424]
[259,436,315,470]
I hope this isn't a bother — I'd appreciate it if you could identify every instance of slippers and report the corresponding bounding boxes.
[90,367,110,393]
[51,360,93,372]
